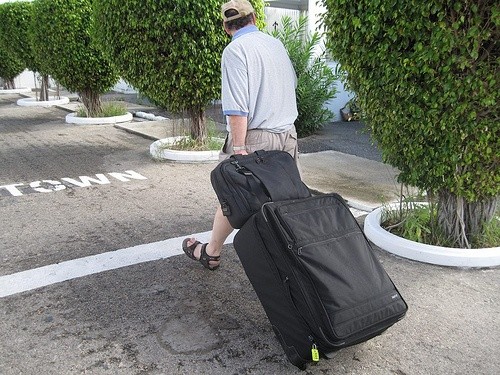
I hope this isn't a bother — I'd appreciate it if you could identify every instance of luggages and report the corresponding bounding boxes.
[233,193,407,369]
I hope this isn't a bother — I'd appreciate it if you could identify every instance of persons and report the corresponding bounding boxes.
[182,0,300,271]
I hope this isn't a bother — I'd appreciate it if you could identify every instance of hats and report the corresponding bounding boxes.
[220,0,255,22]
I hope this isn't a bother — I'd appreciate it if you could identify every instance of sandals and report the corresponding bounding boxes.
[181,238,221,270]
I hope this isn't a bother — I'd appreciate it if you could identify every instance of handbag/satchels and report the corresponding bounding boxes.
[210,149,311,229]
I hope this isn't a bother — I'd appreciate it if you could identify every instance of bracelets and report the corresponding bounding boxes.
[233,146,247,150]
